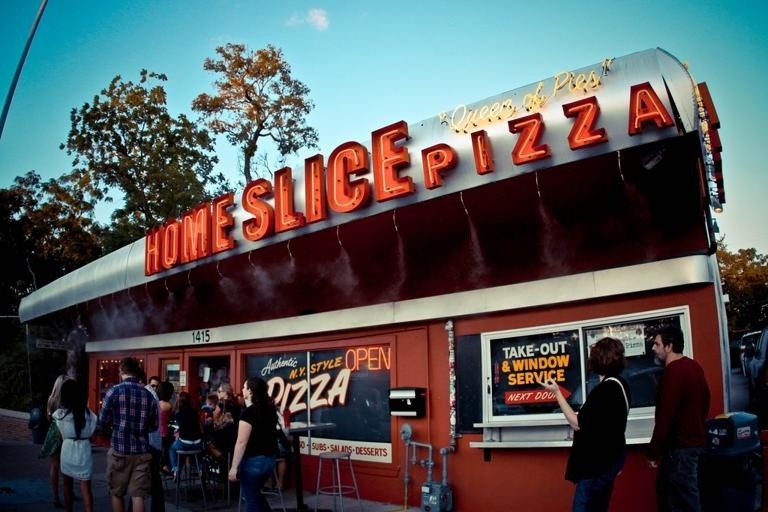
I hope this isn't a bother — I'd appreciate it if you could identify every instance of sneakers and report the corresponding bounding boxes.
[53,496,64,509]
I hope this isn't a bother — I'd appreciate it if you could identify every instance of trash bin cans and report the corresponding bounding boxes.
[28,408,46,444]
[703,412,762,512]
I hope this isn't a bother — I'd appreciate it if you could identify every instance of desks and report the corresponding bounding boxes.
[268,418,336,512]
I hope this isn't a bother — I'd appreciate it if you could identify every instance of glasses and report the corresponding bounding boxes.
[151,384,160,388]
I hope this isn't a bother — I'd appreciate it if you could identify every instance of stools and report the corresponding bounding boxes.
[160,447,286,512]
[313,449,367,512]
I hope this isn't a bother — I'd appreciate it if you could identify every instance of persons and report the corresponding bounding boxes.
[537,337,632,512]
[36,357,286,510]
[647,325,710,511]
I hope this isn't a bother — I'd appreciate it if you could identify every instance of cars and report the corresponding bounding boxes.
[738,325,768,409]
